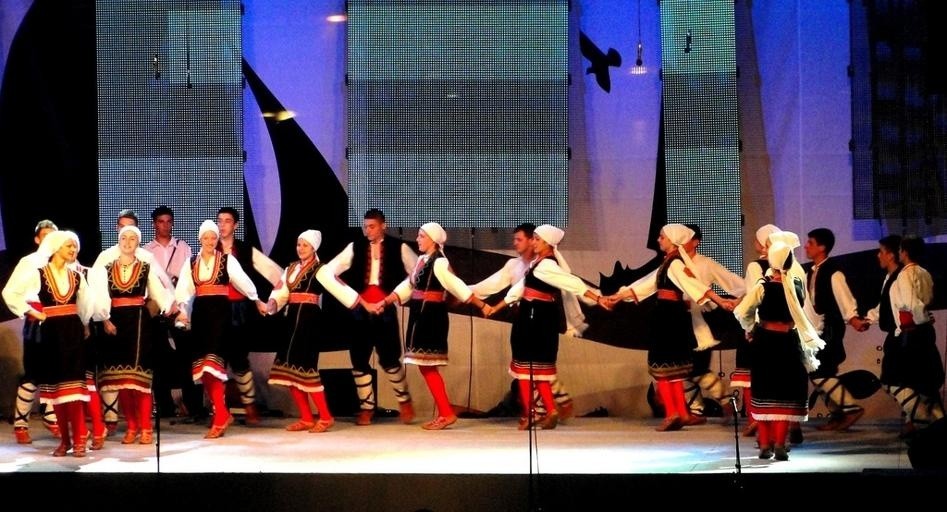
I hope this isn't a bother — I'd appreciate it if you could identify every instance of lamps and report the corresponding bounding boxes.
[630,0,648,76]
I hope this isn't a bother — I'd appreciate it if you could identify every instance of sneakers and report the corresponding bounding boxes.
[90,428,108,450]
[122,429,138,444]
[517,412,539,429]
[45,424,62,438]
[358,410,374,425]
[774,443,789,462]
[13,427,32,443]
[399,404,415,424]
[72,443,85,457]
[204,415,235,438]
[656,415,680,431]
[139,431,154,444]
[758,446,772,460]
[541,409,559,429]
[309,419,335,432]
[285,421,314,431]
[53,443,70,456]
[422,415,459,430]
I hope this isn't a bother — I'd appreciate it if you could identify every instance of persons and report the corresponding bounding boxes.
[682,224,743,424]
[862,234,902,379]
[94,226,179,445]
[759,231,807,445]
[324,210,419,425]
[267,229,384,433]
[10,231,96,458]
[141,206,207,425]
[468,223,604,424]
[733,242,826,460]
[174,219,272,439]
[1,219,60,445]
[376,222,491,431]
[215,207,284,426]
[93,209,175,438]
[608,223,731,433]
[64,231,109,451]
[898,237,945,430]
[491,223,609,432]
[803,228,868,432]
[728,224,799,438]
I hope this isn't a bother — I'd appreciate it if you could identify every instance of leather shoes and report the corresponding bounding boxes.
[836,411,866,432]
